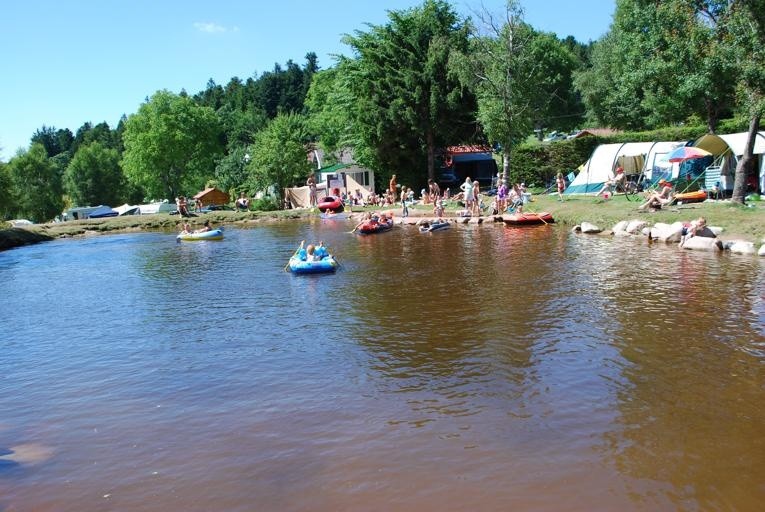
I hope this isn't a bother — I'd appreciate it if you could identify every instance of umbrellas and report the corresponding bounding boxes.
[660,146,713,192]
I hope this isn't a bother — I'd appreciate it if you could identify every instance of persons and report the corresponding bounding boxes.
[235,192,252,213]
[419,177,479,231]
[307,175,414,224]
[595,167,624,197]
[177,195,213,235]
[678,216,707,248]
[638,179,673,213]
[491,178,526,215]
[556,173,565,202]
[299,240,323,262]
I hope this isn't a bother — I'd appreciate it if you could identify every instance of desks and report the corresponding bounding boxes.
[708,189,723,200]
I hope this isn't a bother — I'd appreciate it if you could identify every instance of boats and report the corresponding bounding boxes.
[418,223,451,233]
[286,248,338,275]
[499,211,554,225]
[357,217,394,235]
[317,196,345,212]
[176,228,228,242]
[670,190,706,203]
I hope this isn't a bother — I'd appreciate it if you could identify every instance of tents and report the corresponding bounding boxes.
[562,143,714,195]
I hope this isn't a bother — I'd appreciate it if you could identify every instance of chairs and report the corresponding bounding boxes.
[607,171,631,202]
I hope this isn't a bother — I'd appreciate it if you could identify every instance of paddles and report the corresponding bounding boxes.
[285,240,307,272]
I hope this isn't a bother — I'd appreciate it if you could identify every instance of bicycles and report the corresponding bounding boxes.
[624,167,655,201]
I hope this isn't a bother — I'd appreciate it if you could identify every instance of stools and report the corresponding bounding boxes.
[661,203,672,208]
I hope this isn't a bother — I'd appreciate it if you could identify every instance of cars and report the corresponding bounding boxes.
[7,219,32,228]
[546,130,582,142]
